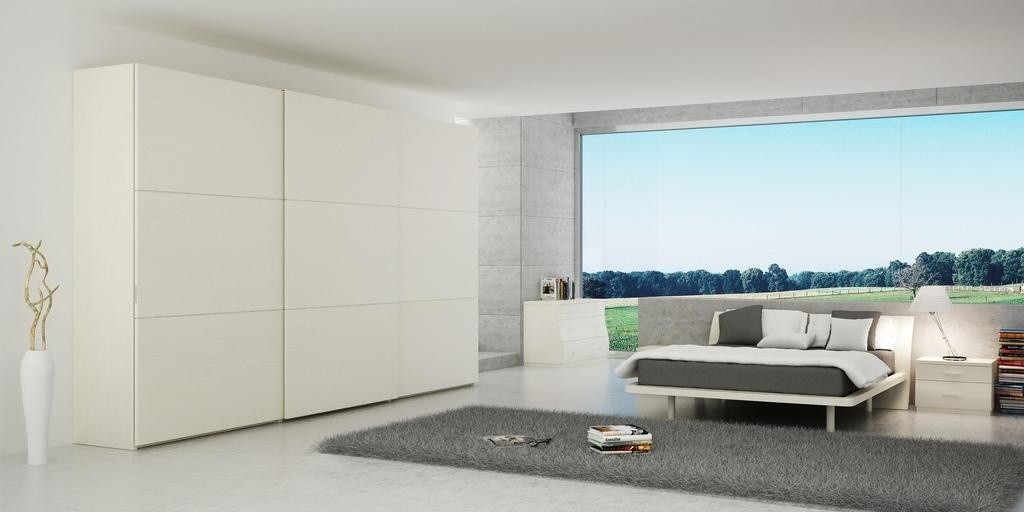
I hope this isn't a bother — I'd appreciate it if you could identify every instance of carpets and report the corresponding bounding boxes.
[317,406,1024,512]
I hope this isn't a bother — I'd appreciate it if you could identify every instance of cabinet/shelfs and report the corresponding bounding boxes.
[523,303,609,368]
[70,62,480,451]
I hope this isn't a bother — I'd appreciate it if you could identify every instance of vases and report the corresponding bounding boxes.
[19,350,53,465]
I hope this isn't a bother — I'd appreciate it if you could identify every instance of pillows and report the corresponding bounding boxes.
[708,305,882,351]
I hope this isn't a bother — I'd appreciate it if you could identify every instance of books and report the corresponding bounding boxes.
[587,423,652,455]
[483,430,526,447]
[996,326,1023,415]
[559,276,574,299]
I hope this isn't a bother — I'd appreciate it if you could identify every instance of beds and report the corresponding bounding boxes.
[624,316,915,434]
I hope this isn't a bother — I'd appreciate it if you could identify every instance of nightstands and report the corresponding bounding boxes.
[915,364,997,416]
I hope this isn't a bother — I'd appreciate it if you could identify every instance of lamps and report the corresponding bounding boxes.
[908,286,966,361]
